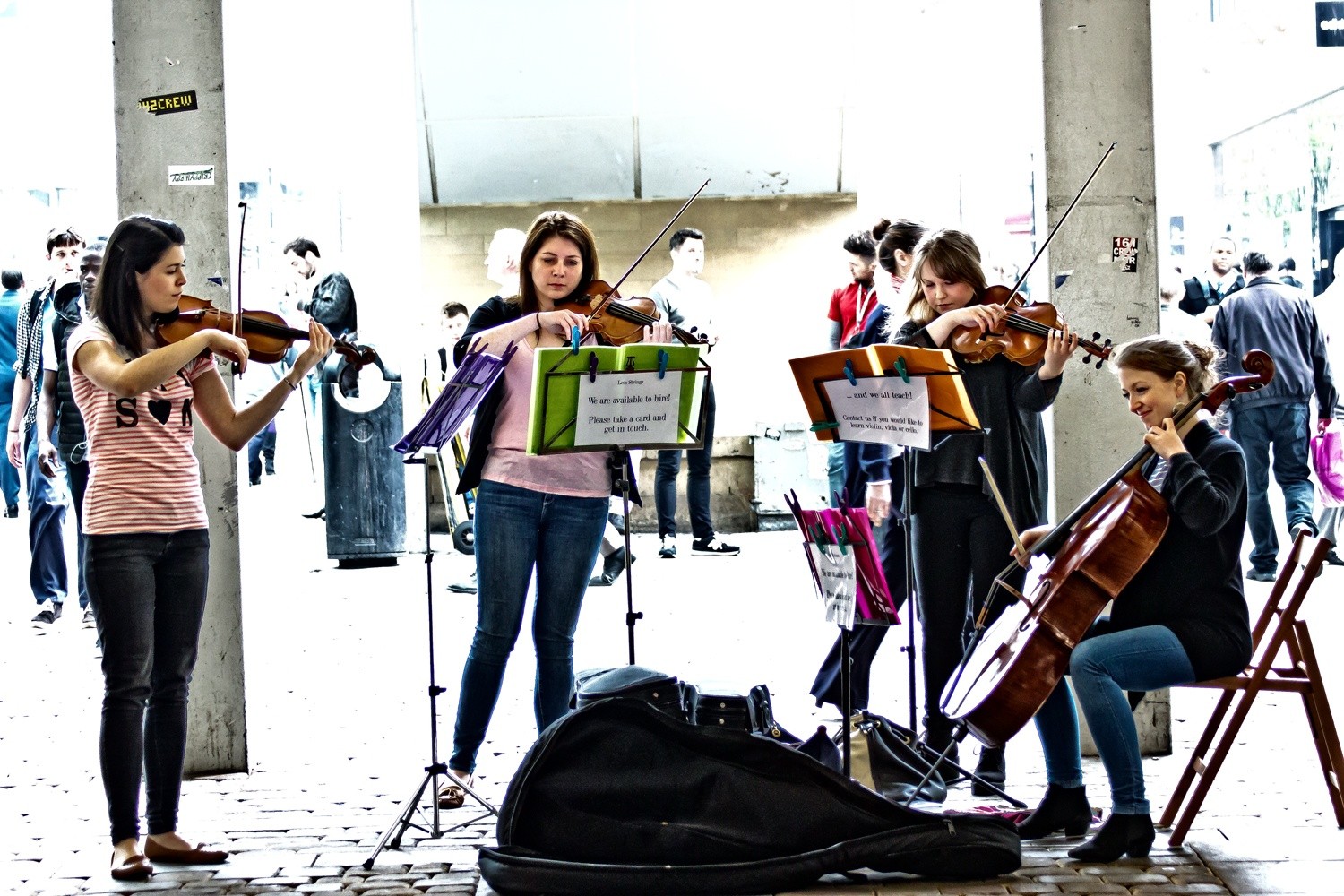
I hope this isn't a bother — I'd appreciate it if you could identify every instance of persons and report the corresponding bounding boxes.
[1008,335,1251,865]
[642,228,742,558]
[0,226,101,632]
[809,218,1078,798]
[284,241,358,522]
[64,218,335,882]
[248,422,274,485]
[438,212,674,806]
[422,303,472,532]
[1156,243,1344,583]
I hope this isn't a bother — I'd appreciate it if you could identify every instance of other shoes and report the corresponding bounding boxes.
[1319,547,1344,566]
[30,598,62,628]
[1246,567,1277,582]
[82,602,96,628]
[1295,526,1323,578]
[658,534,677,559]
[691,532,741,555]
[144,836,229,864]
[608,512,624,534]
[110,849,154,879]
[971,746,1007,796]
[913,729,960,783]
[438,775,474,809]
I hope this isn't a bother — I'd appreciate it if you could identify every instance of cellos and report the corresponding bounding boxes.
[902,347,1276,810]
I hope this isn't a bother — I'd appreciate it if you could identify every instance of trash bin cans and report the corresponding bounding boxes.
[320,369,409,568]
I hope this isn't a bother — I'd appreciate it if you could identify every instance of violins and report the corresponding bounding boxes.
[155,291,376,367]
[552,278,718,354]
[950,284,1112,370]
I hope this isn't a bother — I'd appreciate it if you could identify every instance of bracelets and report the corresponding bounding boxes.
[284,377,296,392]
[536,313,543,332]
[11,429,20,434]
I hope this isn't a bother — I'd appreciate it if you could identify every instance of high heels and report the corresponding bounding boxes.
[1068,812,1156,860]
[1014,785,1092,840]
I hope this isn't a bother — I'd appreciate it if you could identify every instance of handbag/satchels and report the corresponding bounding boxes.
[1310,428,1344,511]
[833,711,947,807]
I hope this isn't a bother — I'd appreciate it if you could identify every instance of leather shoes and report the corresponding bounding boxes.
[588,545,637,587]
[446,566,478,595]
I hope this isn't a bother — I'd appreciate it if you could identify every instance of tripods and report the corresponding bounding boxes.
[363,353,518,868]
[815,372,1030,806]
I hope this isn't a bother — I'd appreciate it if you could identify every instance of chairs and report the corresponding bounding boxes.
[1158,507,1344,847]
